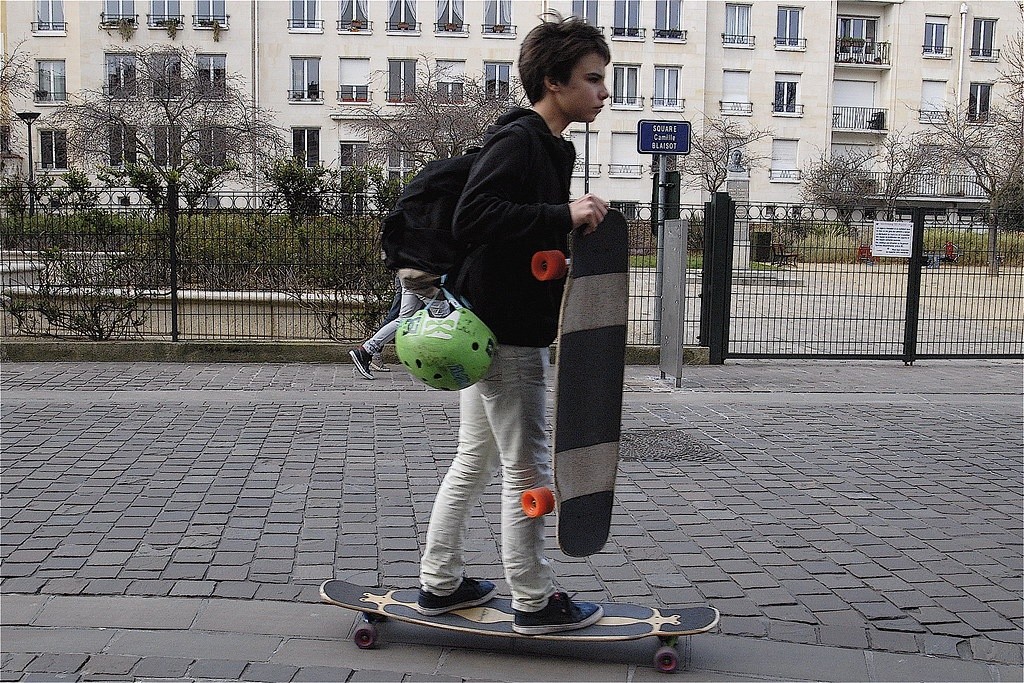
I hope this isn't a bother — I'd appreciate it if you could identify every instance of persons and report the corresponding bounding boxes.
[348,269,451,380]
[417,7,612,634]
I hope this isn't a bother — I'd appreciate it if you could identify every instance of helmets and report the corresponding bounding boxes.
[394,307,498,392]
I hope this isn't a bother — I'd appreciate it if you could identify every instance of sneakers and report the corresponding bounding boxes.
[511,592,604,635]
[368,355,390,371]
[417,577,497,617]
[349,346,374,379]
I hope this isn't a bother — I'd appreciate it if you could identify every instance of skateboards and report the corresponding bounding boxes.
[522,207,632,558]
[319,577,721,674]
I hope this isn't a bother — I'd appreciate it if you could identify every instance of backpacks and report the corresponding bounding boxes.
[377,121,546,277]
